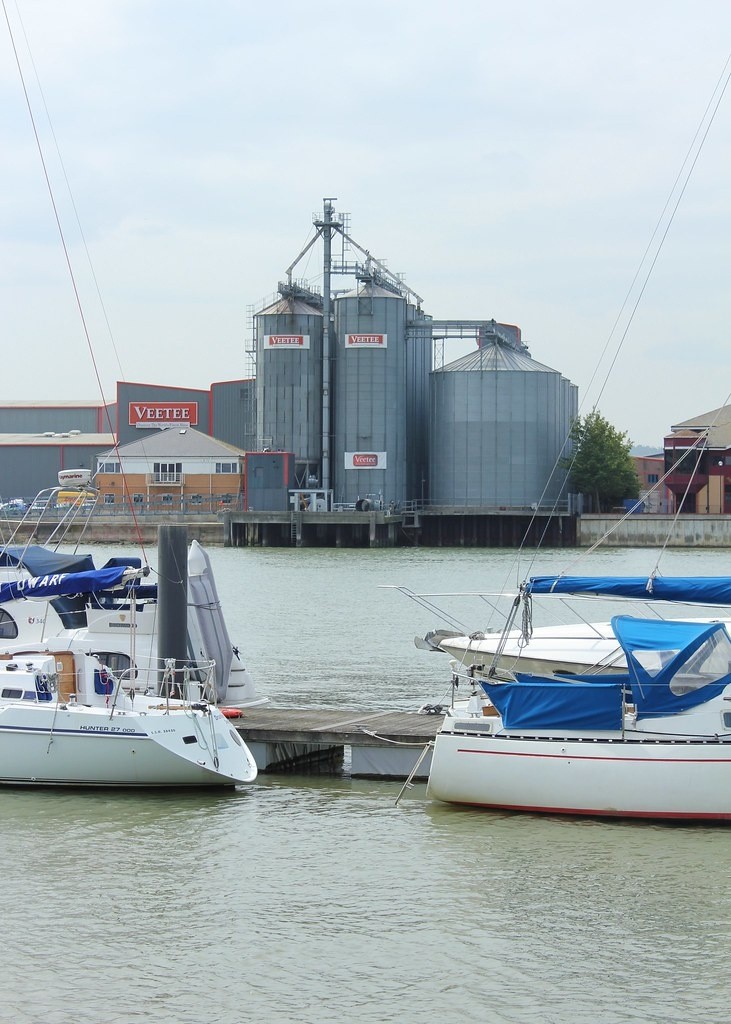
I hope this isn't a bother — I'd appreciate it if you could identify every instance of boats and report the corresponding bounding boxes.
[0,648,259,792]
[388,389,731,823]
[0,440,275,708]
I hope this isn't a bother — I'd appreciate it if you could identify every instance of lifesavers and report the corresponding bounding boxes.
[219,707,243,718]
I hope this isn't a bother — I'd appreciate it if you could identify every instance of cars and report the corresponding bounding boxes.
[0,498,95,522]
